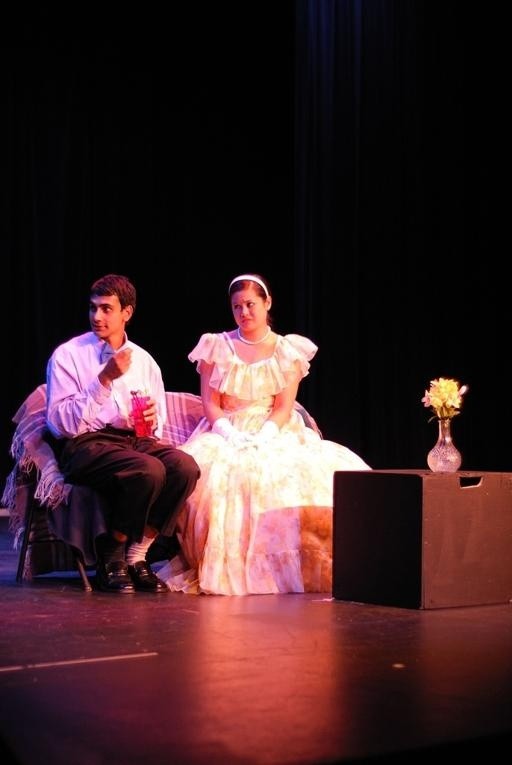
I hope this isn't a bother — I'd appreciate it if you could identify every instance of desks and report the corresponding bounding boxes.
[333,471,512,611]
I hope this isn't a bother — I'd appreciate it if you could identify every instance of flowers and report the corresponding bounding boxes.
[423,378,467,437]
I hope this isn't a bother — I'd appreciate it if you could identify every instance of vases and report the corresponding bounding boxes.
[427,418,462,471]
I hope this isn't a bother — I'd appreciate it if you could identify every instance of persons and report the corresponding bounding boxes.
[44,275,202,595]
[175,274,372,594]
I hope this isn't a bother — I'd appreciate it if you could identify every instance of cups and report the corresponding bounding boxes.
[131,396,153,438]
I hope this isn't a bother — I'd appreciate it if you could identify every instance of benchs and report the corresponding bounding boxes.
[14,392,321,593]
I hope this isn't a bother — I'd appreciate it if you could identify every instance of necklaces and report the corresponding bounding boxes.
[237,325,271,345]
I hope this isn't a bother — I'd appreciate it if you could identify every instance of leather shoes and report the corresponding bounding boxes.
[127,560,169,593]
[95,533,137,594]
